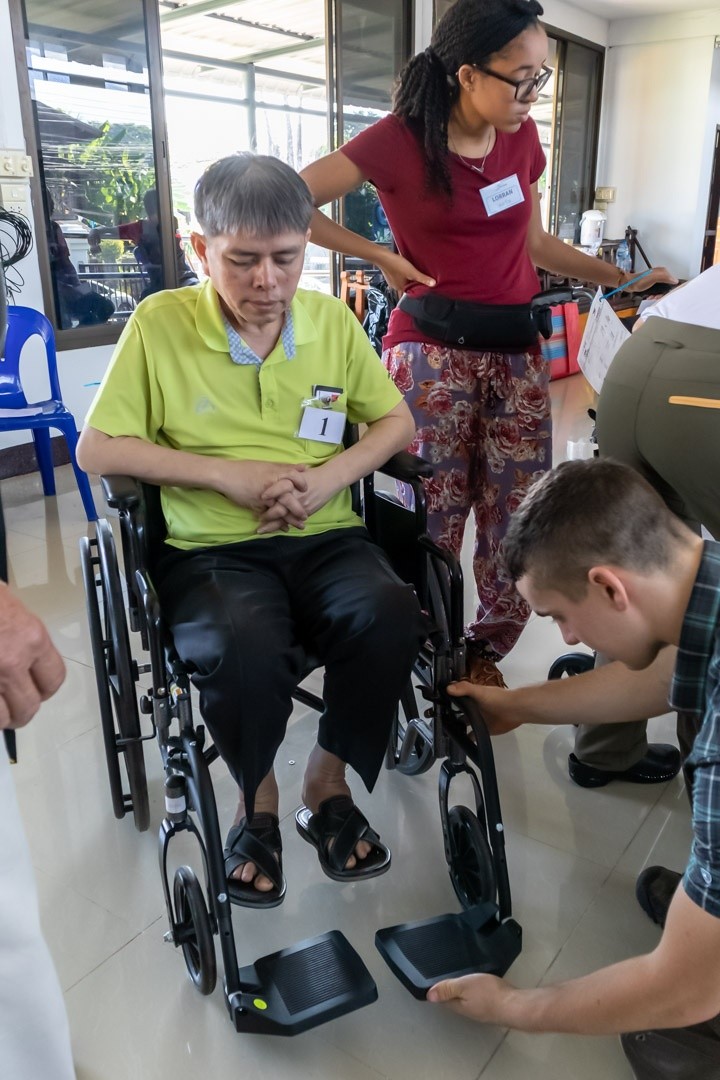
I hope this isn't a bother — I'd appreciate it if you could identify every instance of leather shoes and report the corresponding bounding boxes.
[570,743,681,788]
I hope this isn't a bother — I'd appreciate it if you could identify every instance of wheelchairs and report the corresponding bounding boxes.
[78,418,523,1038]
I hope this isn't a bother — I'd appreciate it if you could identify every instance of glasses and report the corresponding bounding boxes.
[475,64,554,100]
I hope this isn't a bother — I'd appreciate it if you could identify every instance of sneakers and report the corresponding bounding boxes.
[636,866,683,929]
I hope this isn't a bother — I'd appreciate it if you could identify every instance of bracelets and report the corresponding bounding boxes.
[616,266,625,294]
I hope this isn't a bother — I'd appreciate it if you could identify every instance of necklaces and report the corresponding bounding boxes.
[446,127,493,173]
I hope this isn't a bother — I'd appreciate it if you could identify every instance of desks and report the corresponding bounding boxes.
[546,240,624,288]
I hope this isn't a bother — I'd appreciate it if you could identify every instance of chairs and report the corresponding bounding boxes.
[1,303,101,524]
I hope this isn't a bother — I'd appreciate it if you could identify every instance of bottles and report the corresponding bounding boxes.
[616,239,632,273]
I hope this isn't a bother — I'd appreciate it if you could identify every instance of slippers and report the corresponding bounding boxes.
[296,794,392,882]
[224,811,287,909]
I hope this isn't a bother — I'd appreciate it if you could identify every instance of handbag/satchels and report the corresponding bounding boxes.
[576,266,690,395]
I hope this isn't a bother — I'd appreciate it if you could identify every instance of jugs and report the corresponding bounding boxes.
[579,210,607,246]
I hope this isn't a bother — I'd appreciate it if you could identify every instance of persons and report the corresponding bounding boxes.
[77,154,425,909]
[0,493,77,1080]
[296,0,678,688]
[428,460,720,1034]
[46,187,115,330]
[88,189,199,302]
[569,263,720,788]
